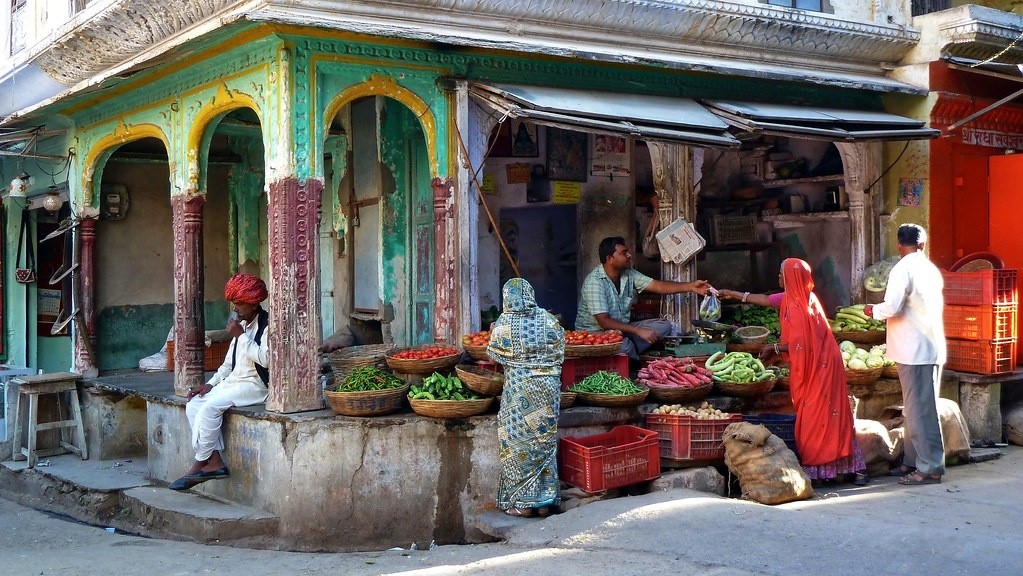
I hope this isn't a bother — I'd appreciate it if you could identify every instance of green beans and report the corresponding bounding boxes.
[567,369,645,396]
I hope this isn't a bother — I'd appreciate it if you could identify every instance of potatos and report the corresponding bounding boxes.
[569,400,730,484]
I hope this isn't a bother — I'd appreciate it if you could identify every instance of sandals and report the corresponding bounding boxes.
[897,470,941,484]
[888,464,918,476]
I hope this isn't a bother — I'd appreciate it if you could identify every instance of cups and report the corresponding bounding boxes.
[227,310,240,323]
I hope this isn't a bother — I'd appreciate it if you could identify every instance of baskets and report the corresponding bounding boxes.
[736,325,771,345]
[565,384,650,407]
[405,393,496,419]
[454,364,505,394]
[167,341,231,371]
[956,259,993,272]
[384,345,463,385]
[644,381,713,403]
[884,365,900,379]
[843,367,883,384]
[778,375,791,391]
[559,392,577,410]
[324,378,410,416]
[713,379,777,397]
[830,330,886,345]
[462,343,492,361]
[563,342,623,359]
[328,343,399,382]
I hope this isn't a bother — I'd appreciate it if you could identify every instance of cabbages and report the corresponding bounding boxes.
[838,340,895,370]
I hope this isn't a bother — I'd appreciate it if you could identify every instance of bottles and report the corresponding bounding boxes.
[320,373,328,407]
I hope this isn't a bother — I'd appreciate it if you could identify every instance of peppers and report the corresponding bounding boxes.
[336,366,485,402]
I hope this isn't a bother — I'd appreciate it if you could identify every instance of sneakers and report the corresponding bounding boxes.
[855,468,870,486]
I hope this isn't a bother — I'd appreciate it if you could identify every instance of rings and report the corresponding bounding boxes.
[189,387,192,392]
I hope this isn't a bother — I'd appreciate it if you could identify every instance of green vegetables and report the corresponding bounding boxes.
[725,306,780,345]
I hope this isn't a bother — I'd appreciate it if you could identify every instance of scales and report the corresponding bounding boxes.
[664,319,739,357]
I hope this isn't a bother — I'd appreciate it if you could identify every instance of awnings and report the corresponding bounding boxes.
[467,82,742,151]
[701,98,942,143]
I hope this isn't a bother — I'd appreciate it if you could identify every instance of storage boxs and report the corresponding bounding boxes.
[742,411,798,456]
[534,179,587,205]
[941,301,1019,341]
[165,339,230,372]
[560,424,661,492]
[943,338,1017,378]
[646,412,742,462]
[702,214,758,248]
[938,268,1019,306]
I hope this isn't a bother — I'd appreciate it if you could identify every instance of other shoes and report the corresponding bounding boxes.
[169,476,199,490]
[184,466,230,479]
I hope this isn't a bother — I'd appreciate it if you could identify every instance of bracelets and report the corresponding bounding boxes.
[742,292,751,303]
[869,306,873,318]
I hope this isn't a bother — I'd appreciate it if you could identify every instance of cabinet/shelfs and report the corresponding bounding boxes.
[762,173,851,229]
[12,372,88,468]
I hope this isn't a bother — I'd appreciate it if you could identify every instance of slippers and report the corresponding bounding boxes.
[504,506,550,518]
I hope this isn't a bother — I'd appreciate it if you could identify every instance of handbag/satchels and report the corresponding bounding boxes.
[699,288,721,324]
[15,268,37,283]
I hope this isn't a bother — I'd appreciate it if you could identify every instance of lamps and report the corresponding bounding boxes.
[44,171,63,214]
[8,158,28,199]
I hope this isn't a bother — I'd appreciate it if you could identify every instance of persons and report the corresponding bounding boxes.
[574,236,713,382]
[713,258,868,488]
[863,222,947,486]
[485,277,565,517]
[168,273,269,490]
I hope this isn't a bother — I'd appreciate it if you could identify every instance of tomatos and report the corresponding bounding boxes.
[390,329,623,360]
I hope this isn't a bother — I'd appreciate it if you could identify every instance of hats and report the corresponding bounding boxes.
[224,273,268,304]
